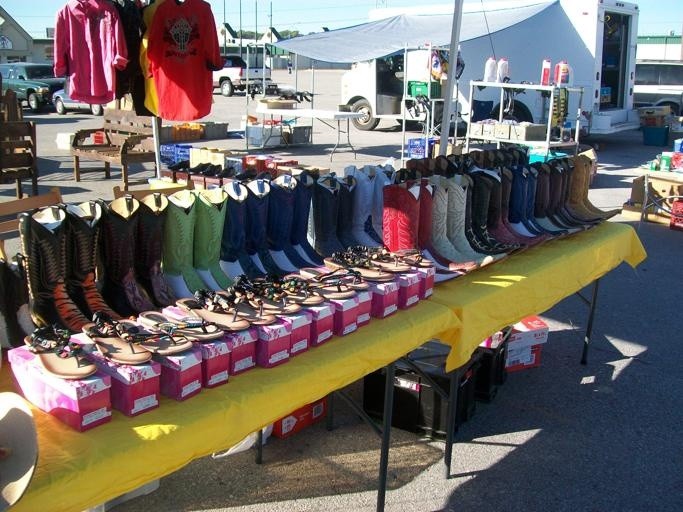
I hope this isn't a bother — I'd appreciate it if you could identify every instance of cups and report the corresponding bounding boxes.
[562,121,571,143]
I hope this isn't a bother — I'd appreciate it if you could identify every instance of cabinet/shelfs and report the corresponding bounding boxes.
[399,41,461,169]
[464,80,583,163]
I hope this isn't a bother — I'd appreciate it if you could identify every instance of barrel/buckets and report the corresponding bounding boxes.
[554,59,574,87]
[484,57,497,82]
[496,58,509,82]
[541,59,550,84]
[294,126,311,143]
[264,126,280,146]
[247,125,262,145]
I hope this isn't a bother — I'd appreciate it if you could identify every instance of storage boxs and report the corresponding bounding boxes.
[247,123,311,147]
[259,315,291,367]
[637,106,671,146]
[159,122,229,144]
[331,294,359,336]
[410,264,434,300]
[73,327,162,420]
[316,267,371,327]
[222,327,257,373]
[470,122,546,142]
[305,302,336,344]
[408,138,463,159]
[393,272,421,309]
[123,314,204,402]
[160,168,237,191]
[369,281,400,318]
[5,334,113,432]
[408,78,448,99]
[161,301,232,389]
[282,312,312,355]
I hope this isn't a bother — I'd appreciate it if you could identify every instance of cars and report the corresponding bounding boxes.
[0,61,105,116]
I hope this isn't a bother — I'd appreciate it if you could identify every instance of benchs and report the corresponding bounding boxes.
[69,109,162,191]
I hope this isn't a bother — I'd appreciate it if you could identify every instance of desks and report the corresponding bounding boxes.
[634,158,682,224]
[0,301,453,512]
[255,107,364,163]
[425,219,633,512]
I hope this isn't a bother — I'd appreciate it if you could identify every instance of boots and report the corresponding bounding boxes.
[382,149,622,283]
[9,163,396,348]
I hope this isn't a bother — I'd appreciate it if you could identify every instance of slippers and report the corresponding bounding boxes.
[24,245,435,379]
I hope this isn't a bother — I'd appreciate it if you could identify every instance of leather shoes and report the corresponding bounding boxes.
[168,160,271,180]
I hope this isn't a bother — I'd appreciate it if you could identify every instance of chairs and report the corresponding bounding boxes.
[112,180,195,200]
[0,117,64,260]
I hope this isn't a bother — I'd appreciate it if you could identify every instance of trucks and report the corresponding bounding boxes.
[339,0,641,135]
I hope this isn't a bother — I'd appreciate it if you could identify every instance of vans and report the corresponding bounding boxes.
[634,59,683,115]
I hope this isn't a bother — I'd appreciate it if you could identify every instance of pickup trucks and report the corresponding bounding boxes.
[212,55,272,97]
